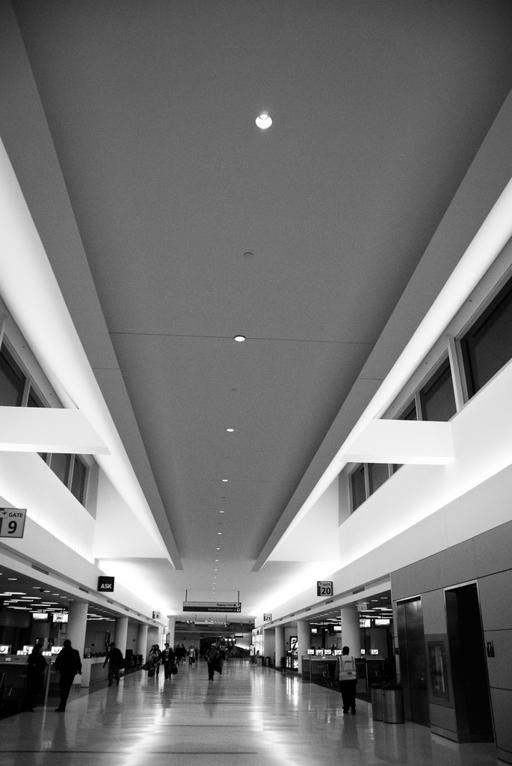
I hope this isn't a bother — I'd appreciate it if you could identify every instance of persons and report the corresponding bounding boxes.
[334,646,358,714]
[26,644,46,708]
[54,639,83,711]
[103,642,124,687]
[206,643,219,681]
[146,643,198,680]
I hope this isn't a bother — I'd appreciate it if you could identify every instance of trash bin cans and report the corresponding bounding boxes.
[257,656,264,667]
[135,654,143,669]
[371,681,402,724]
[126,649,133,669]
[265,657,270,667]
